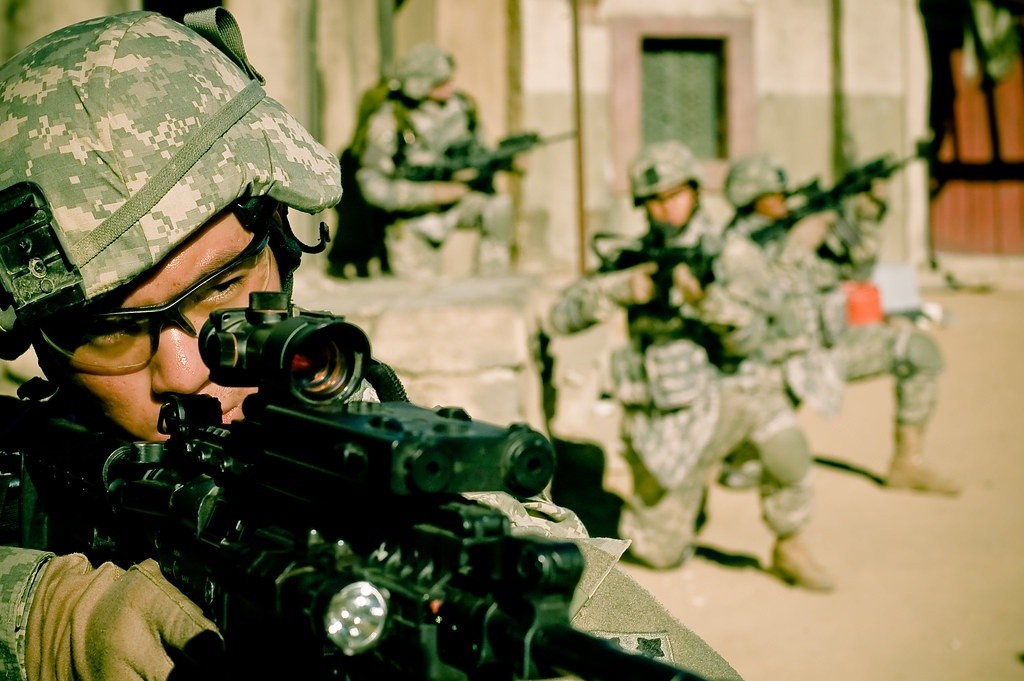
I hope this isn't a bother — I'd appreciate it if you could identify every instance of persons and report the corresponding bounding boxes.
[548,137,837,592]
[358,43,521,277]
[0,6,587,681]
[718,151,966,496]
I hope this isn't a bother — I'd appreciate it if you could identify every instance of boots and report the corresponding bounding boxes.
[886,425,967,492]
[769,536,845,589]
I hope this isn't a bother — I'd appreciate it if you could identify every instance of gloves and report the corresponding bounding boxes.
[630,261,658,305]
[24,537,223,681]
[672,262,705,306]
[787,207,839,249]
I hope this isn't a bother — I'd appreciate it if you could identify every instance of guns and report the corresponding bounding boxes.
[605,232,723,316]
[0,288,712,681]
[752,137,933,260]
[393,132,569,195]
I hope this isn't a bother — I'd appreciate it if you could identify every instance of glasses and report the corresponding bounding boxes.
[38,222,271,372]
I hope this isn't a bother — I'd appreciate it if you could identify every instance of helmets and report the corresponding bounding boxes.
[0,11,343,361]
[628,140,709,207]
[723,153,787,210]
[393,44,455,100]
